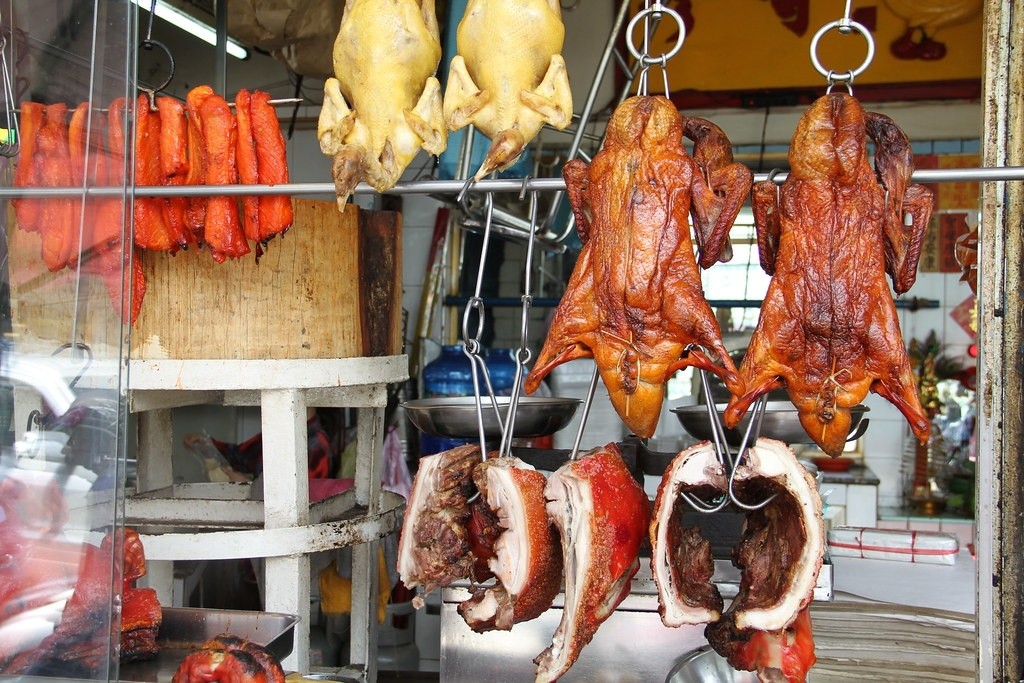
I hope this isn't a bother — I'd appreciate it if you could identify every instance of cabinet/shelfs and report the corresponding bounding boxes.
[8,353,410,683]
